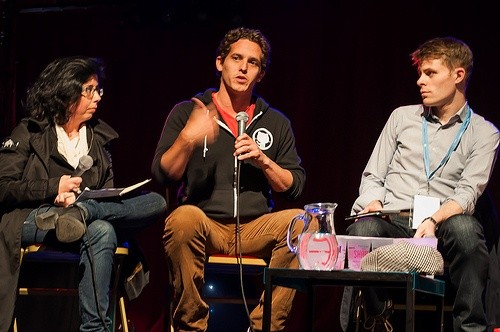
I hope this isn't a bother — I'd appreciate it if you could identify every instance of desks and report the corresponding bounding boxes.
[262,268,445,332]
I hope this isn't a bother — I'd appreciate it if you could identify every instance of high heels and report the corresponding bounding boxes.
[35,206,88,243]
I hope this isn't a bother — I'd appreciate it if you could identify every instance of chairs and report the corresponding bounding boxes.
[165,184,270,332]
[11,243,130,332]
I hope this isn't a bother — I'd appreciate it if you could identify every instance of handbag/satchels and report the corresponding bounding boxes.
[359,240,445,276]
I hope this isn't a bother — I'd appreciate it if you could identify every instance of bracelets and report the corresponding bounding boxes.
[422,217,439,231]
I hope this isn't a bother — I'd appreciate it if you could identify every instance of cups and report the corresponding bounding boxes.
[298,234,439,279]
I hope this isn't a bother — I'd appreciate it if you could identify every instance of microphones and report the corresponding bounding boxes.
[70,154,93,191]
[235,111,249,156]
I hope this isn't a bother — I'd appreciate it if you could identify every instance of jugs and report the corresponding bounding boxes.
[287,202,338,271]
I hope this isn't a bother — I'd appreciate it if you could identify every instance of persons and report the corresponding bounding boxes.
[341,37,500,332]
[0,57,166,332]
[151,28,319,331]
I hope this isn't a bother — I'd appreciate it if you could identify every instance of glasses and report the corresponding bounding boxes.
[80,87,104,98]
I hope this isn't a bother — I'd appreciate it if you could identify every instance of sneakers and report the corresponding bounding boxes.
[363,299,394,332]
